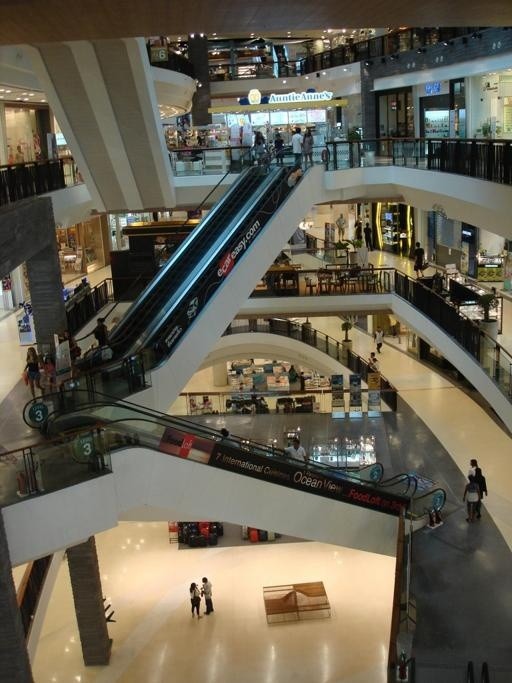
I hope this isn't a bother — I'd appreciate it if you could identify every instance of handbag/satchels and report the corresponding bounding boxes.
[467,492,479,502]
[192,596,201,606]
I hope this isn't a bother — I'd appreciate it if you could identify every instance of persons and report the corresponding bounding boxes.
[335,213,347,242]
[249,130,267,167]
[271,133,286,166]
[374,325,385,353]
[220,428,240,449]
[248,358,255,370]
[189,582,203,619]
[288,365,308,390]
[15,145,25,164]
[190,398,212,411]
[23,346,45,405]
[411,241,426,279]
[276,438,308,469]
[466,457,485,482]
[369,350,380,367]
[42,355,58,394]
[473,466,488,518]
[63,330,79,365]
[291,127,305,169]
[392,325,401,344]
[461,473,482,522]
[250,385,257,400]
[239,382,244,398]
[238,369,244,382]
[197,136,202,146]
[301,125,314,170]
[92,316,112,347]
[199,576,214,615]
[355,220,362,241]
[367,356,379,370]
[362,221,374,252]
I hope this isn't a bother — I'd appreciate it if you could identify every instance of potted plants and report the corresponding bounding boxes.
[341,322,352,350]
[333,239,364,269]
[476,294,499,342]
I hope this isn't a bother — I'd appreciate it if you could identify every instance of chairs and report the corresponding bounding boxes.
[305,263,383,293]
